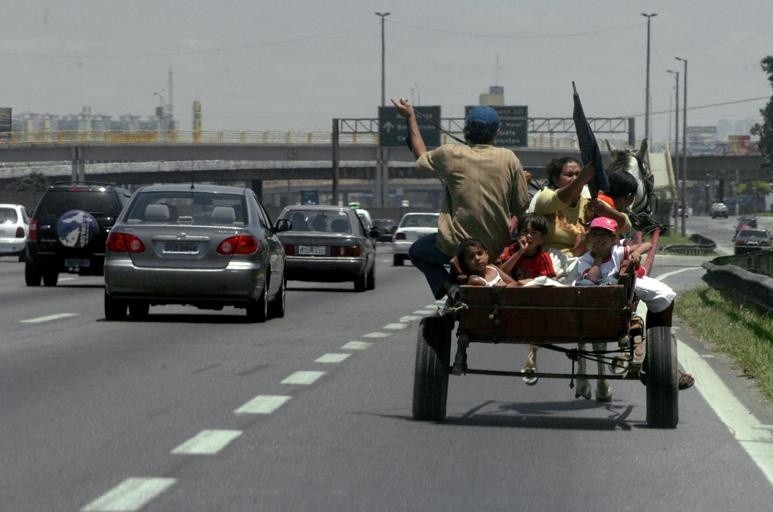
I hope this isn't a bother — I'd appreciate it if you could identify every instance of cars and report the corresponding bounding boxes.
[0,202,32,261]
[351,206,399,244]
[389,211,442,266]
[102,182,292,323]
[672,201,772,255]
[270,202,381,294]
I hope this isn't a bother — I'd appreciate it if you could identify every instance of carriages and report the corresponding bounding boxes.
[410,136,682,430]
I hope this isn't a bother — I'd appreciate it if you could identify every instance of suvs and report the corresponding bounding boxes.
[21,180,133,287]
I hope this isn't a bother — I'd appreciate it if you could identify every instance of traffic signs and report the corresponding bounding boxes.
[463,104,528,148]
[377,104,442,150]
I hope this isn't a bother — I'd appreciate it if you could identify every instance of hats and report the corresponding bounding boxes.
[464,106,501,142]
[589,217,619,235]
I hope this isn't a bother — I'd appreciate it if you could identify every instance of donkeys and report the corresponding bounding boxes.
[572,137,657,401]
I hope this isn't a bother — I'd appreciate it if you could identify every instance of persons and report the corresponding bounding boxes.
[388,95,696,388]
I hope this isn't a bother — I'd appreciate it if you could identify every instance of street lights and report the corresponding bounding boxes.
[373,11,392,207]
[664,56,688,237]
[638,12,657,143]
